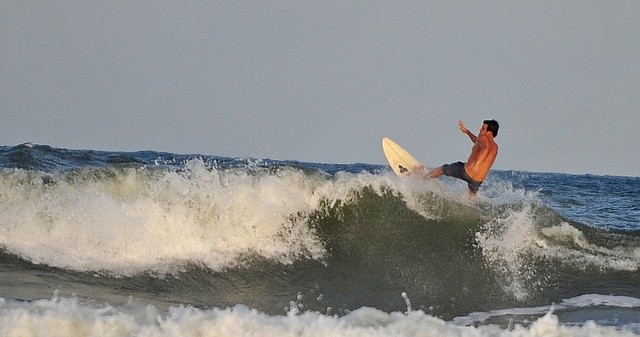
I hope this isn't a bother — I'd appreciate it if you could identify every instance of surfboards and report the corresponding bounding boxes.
[382,137,429,180]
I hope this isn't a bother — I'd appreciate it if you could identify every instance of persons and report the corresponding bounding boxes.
[415,119,500,196]
